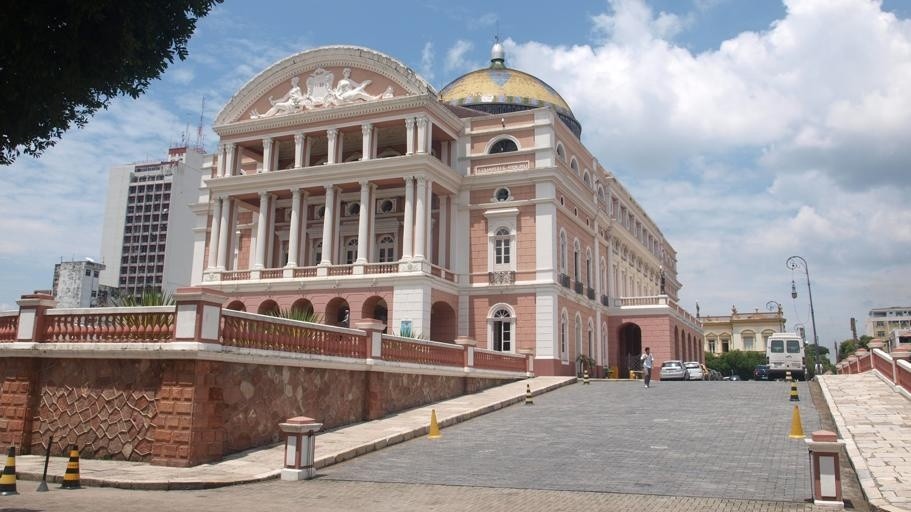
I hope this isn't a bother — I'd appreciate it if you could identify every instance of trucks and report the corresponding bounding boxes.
[765,331,809,380]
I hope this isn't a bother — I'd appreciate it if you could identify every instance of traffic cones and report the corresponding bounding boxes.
[522,382,535,407]
[58,444,85,491]
[425,408,443,440]
[582,369,592,385]
[789,378,801,402]
[0,446,21,497]
[783,362,794,381]
[785,403,810,439]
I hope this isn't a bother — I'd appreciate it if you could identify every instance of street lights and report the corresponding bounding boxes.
[765,300,784,333]
[785,255,821,375]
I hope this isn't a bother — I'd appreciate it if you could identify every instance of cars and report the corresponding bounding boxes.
[684,360,742,381]
[658,359,692,381]
[751,364,769,380]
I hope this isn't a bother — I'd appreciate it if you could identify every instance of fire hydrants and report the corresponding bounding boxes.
[628,369,636,381]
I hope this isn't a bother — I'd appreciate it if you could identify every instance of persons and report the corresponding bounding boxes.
[258,76,301,120]
[640,347,654,388]
[334,67,383,108]
